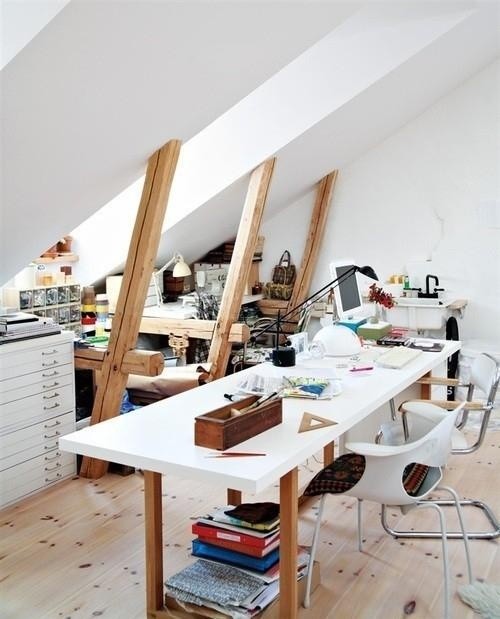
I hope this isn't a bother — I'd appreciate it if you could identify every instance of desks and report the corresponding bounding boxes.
[56,334,466,617]
[147,289,264,328]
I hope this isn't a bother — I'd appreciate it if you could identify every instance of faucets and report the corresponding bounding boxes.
[426,275,439,294]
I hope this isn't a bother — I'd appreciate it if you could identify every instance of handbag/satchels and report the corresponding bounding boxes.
[273,250,295,285]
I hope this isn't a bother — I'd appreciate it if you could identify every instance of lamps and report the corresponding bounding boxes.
[152,253,192,311]
[250,265,379,366]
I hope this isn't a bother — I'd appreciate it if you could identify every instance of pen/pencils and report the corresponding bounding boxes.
[240,391,281,413]
[207,452,267,457]
[350,368,373,371]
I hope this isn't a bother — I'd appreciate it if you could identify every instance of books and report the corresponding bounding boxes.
[192,505,282,559]
[408,342,444,352]
[376,335,411,346]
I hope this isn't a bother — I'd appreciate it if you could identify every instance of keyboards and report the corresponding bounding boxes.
[374,344,423,370]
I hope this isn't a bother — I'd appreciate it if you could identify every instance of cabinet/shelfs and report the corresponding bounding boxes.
[0,331,79,510]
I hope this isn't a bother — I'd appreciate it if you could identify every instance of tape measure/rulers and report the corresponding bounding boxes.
[297,411,338,433]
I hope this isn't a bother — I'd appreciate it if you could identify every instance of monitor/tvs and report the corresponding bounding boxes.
[329,259,364,321]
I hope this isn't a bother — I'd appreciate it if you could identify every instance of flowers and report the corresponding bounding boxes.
[368,283,399,310]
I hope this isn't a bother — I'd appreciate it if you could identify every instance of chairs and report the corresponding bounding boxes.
[380,350,500,542]
[298,398,474,617]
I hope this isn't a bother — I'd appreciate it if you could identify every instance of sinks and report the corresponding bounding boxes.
[388,298,449,330]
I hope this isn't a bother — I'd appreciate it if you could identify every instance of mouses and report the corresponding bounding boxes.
[413,340,435,348]
